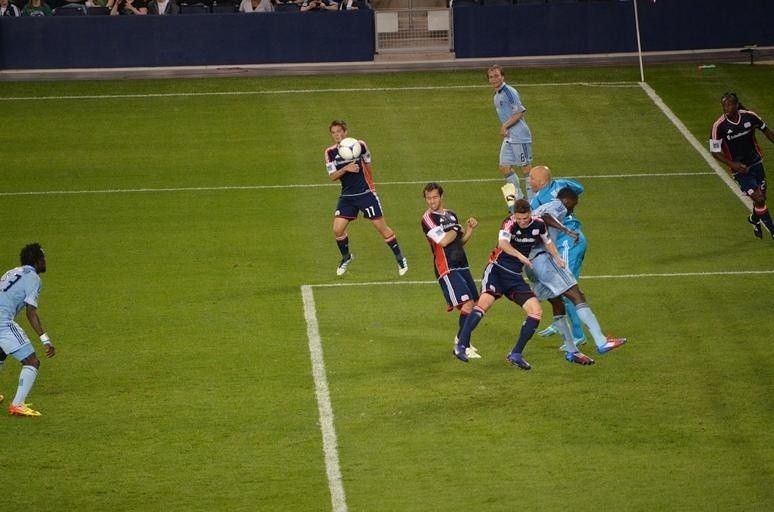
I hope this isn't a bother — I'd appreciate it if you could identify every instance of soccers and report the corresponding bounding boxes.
[338,137,362,161]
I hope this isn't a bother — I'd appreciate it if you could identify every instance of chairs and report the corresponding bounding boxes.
[53,4,299,15]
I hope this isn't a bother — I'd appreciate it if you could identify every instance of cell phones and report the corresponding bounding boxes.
[315,2,321,7]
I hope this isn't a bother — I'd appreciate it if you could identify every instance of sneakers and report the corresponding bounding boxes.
[505,351,532,370]
[564,351,595,366]
[452,334,482,362]
[0,394,43,417]
[397,257,408,277]
[336,253,352,275]
[596,333,627,354]
[538,323,560,337]
[748,214,762,238]
[760,212,774,236]
[560,333,588,351]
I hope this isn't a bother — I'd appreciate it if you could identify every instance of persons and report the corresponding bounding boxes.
[421,182,482,359]
[500,164,587,353]
[525,188,627,365]
[325,120,409,277]
[709,92,774,240]
[487,63,536,200]
[1,1,375,17]
[453,200,566,369]
[0,242,55,417]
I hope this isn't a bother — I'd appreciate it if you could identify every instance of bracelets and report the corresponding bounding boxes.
[39,333,51,344]
